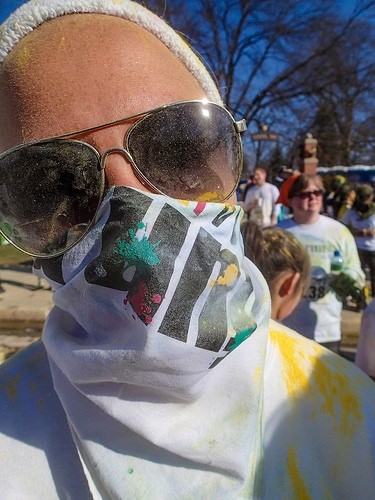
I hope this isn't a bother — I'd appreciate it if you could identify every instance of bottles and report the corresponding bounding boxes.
[330,250,343,275]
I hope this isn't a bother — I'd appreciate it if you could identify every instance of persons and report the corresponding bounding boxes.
[236,168,375,381]
[0,0,375,500]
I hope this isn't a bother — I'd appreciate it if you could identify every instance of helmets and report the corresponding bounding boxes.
[355,183,374,203]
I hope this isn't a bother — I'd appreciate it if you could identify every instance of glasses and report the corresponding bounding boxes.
[290,189,323,199]
[0,99,247,259]
